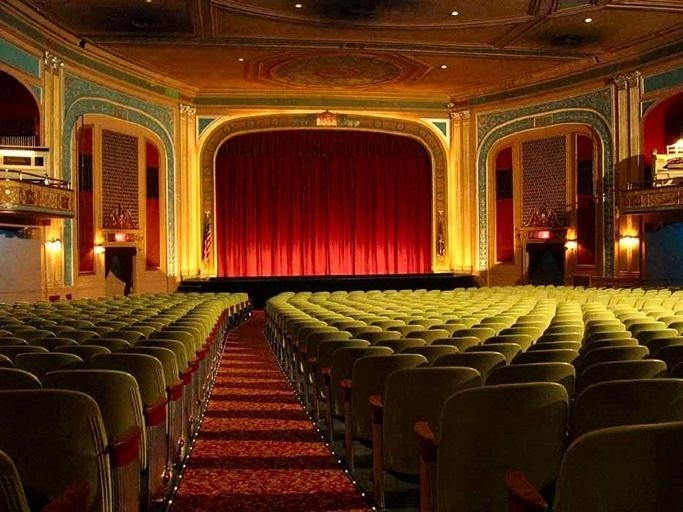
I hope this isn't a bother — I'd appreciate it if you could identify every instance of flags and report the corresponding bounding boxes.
[437,215,445,257]
[201,220,213,267]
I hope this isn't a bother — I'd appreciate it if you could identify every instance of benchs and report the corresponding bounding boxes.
[649,139,682,187]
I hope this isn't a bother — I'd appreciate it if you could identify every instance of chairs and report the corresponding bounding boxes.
[266,283,682,512]
[1,293,249,511]
